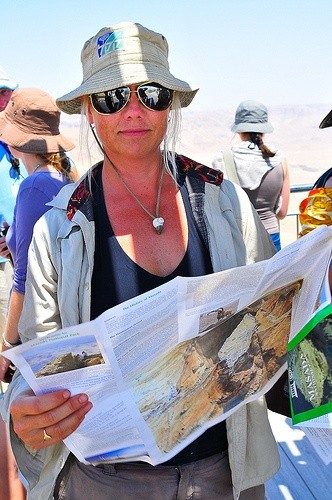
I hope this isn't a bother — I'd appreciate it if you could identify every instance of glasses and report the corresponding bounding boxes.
[88,82,177,116]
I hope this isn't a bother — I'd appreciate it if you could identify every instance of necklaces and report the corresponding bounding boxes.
[33,164,40,172]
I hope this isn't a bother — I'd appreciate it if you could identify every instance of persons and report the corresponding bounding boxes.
[0,22,277,500]
[211,103,290,251]
[0,78,79,500]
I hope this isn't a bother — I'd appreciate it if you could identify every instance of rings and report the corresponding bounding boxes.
[43,427,53,439]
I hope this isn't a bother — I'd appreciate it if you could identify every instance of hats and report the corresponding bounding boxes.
[57,22,200,115]
[0,87,75,154]
[230,101,274,134]
